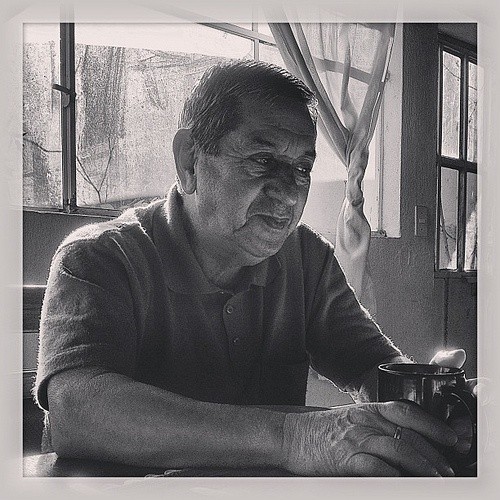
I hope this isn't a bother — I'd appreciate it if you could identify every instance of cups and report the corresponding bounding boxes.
[378,363,477,464]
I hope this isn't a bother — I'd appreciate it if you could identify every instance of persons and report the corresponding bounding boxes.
[32,61,478,478]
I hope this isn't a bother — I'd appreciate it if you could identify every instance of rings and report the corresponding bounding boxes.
[394,426,403,440]
